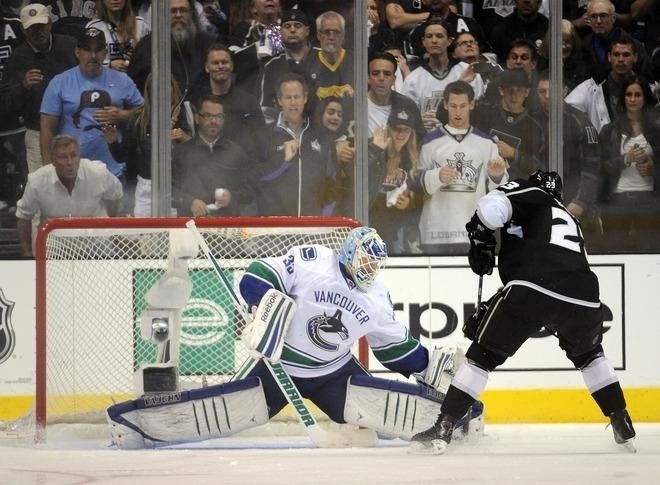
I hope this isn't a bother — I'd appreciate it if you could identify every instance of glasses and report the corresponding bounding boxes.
[197,111,225,120]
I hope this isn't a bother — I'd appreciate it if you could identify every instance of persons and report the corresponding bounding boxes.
[345,0,402,58]
[250,73,341,217]
[488,0,550,73]
[615,0,660,33]
[580,0,653,82]
[106,225,483,451]
[330,110,422,257]
[259,9,323,123]
[563,35,660,133]
[410,169,637,455]
[172,95,258,259]
[526,68,605,226]
[0,17,24,80]
[37,0,98,41]
[304,11,355,139]
[38,27,144,217]
[335,52,428,164]
[312,97,356,165]
[0,0,33,24]
[101,71,193,217]
[127,0,229,92]
[383,45,411,94]
[401,17,484,132]
[230,0,286,105]
[190,45,266,168]
[403,2,498,69]
[84,0,151,72]
[16,135,124,259]
[4,4,82,257]
[414,82,509,255]
[383,1,457,50]
[477,39,547,131]
[557,0,627,24]
[535,19,589,88]
[489,0,548,60]
[597,76,660,254]
[449,30,504,78]
[436,62,546,182]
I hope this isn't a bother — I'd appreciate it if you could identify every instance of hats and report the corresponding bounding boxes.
[77,27,108,53]
[499,68,533,88]
[280,8,310,26]
[387,106,418,128]
[19,3,50,30]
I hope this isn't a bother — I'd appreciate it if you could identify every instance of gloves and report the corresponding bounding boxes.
[416,345,465,395]
[466,214,497,275]
[240,289,298,363]
[463,291,498,341]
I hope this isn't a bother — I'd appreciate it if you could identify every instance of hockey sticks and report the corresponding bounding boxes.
[185,219,379,448]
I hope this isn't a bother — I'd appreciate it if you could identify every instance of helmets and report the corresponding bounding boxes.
[530,169,563,198]
[337,226,389,294]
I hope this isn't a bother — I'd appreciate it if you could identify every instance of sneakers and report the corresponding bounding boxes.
[601,403,636,445]
[406,421,451,444]
